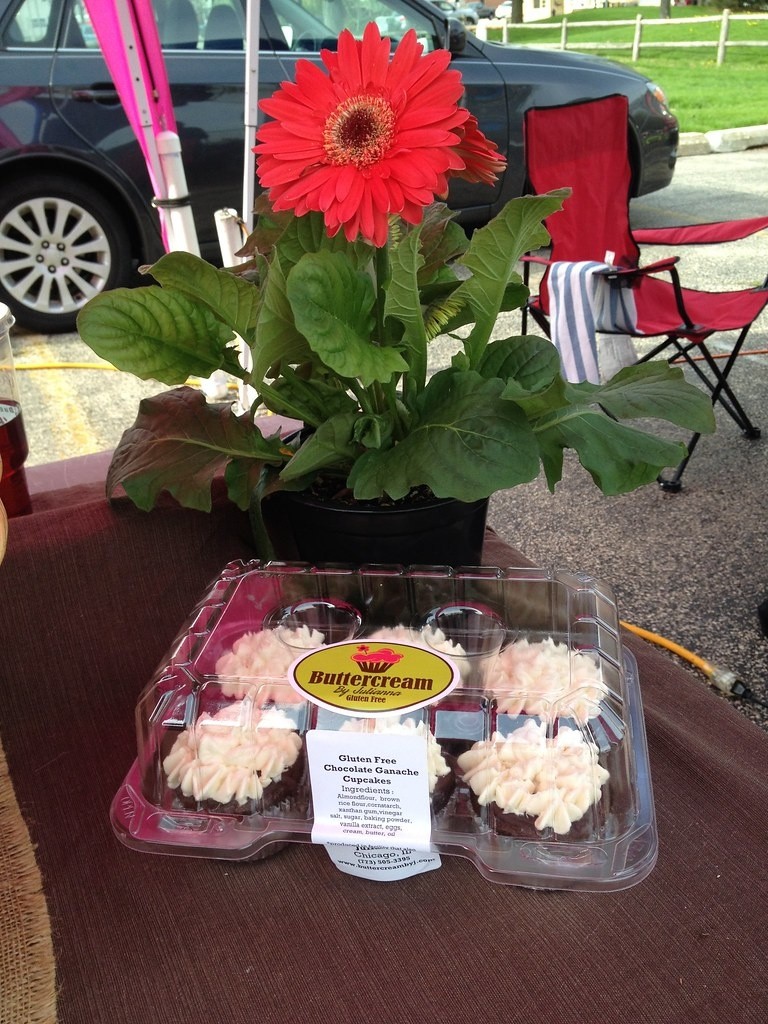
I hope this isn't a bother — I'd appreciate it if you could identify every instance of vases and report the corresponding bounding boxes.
[266,424,488,568]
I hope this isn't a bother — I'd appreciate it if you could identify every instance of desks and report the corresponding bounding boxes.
[0,445,768,1024]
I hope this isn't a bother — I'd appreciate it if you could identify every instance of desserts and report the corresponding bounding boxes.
[368,627,471,704]
[339,719,452,812]
[456,720,610,838]
[214,627,326,706]
[161,699,302,816]
[478,638,607,732]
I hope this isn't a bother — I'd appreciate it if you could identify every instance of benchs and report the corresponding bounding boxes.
[159,0,201,50]
[204,4,243,51]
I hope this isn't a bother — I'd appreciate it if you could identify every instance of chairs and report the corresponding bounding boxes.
[516,91,768,493]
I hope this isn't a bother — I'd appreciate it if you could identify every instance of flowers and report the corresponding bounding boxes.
[75,19,718,563]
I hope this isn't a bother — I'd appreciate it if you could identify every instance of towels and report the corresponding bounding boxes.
[546,259,638,385]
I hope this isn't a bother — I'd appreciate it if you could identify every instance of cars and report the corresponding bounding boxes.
[0,1,682,335]
[494,1,516,20]
[431,0,480,28]
[457,1,494,21]
